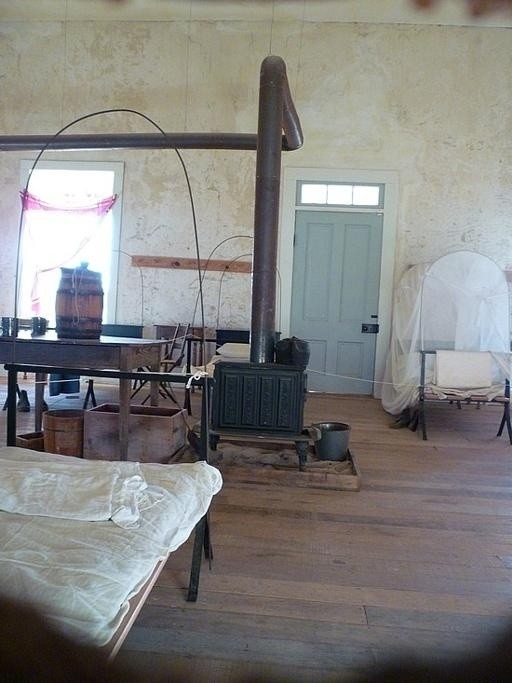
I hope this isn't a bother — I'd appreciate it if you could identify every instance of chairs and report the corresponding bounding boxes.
[130,321,191,405]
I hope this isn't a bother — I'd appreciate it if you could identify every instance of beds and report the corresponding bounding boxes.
[0,364,217,667]
[388,247,512,442]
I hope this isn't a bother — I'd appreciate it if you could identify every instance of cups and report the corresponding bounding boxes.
[1,316,20,337]
[31,315,50,335]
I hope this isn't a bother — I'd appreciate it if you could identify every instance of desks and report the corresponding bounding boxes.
[0,328,171,460]
[153,323,208,370]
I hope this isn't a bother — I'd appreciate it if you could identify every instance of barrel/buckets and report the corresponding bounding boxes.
[54,261,104,339]
[314,420,352,461]
[42,409,85,459]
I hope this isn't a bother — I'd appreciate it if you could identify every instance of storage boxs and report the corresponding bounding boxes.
[83,400,188,463]
